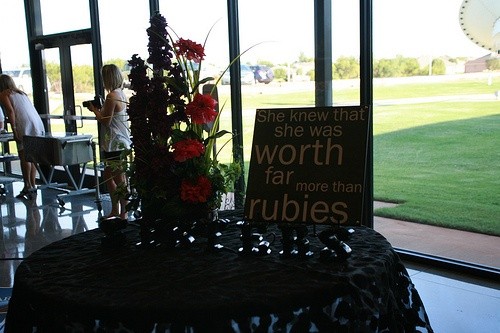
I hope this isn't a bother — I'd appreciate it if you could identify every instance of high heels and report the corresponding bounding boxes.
[15,187,38,200]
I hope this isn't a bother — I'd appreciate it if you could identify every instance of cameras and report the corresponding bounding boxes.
[82,95,104,108]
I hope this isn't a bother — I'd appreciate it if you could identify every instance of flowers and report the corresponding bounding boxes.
[88,9,269,222]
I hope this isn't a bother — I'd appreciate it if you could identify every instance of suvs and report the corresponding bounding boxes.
[220,64,273,84]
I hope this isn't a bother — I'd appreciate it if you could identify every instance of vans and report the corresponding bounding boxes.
[1,68,51,95]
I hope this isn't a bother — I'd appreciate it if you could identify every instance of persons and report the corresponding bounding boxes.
[0,74,45,198]
[87,64,131,219]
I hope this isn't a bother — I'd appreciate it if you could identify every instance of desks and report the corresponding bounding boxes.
[23,133,96,206]
[5,209,435,333]
